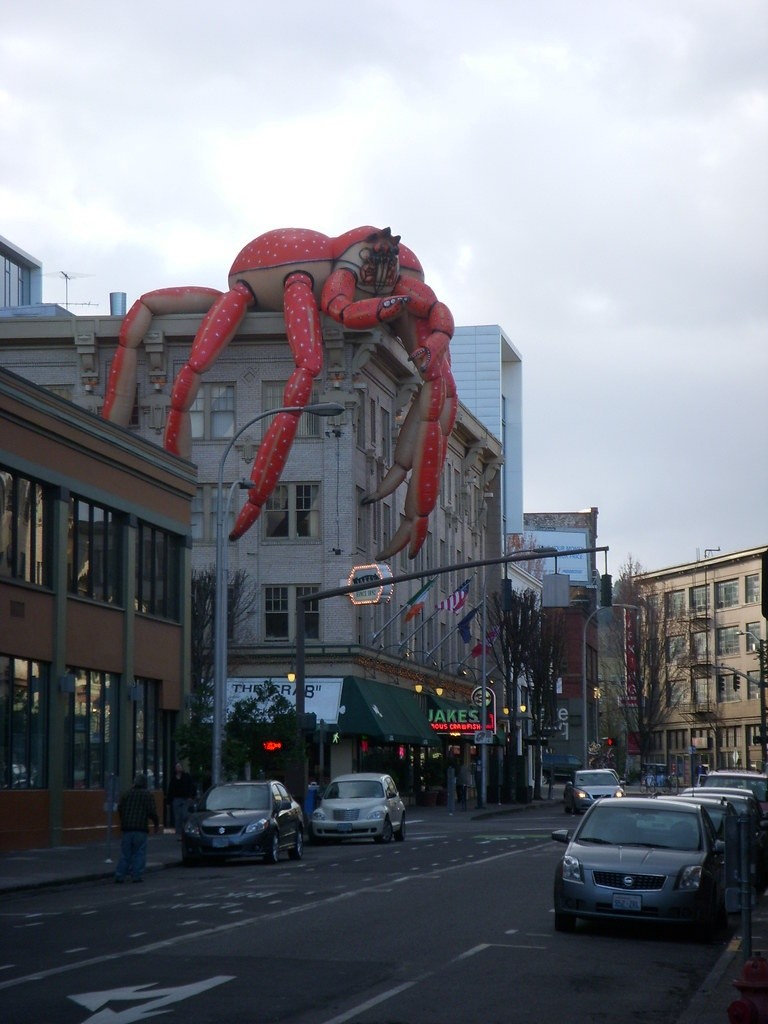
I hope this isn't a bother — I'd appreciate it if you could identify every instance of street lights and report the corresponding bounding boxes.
[581,605,637,769]
[690,663,767,771]
[479,546,559,808]
[210,401,347,775]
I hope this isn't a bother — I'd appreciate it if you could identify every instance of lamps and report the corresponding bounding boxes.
[84,377,93,390]
[287,637,297,682]
[334,371,341,387]
[373,645,526,714]
[154,375,161,389]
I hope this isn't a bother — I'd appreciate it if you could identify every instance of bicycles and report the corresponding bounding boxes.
[640,783,655,794]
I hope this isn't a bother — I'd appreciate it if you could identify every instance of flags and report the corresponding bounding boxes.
[471,625,502,658]
[457,605,481,641]
[404,580,436,622]
[433,577,472,616]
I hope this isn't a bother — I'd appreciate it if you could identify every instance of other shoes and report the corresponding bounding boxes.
[177,834,183,840]
[114,879,124,883]
[133,880,143,883]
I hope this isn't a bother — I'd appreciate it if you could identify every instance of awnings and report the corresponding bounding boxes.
[338,676,444,744]
[426,694,508,745]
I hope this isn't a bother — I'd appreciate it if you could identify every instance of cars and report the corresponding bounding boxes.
[655,767,768,908]
[179,778,305,865]
[308,772,407,845]
[551,796,726,941]
[564,767,627,815]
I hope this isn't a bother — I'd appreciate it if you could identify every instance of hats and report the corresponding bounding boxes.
[135,775,145,785]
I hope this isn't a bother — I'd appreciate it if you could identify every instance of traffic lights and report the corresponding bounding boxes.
[328,731,341,744]
[718,676,725,693]
[732,675,740,691]
[607,737,616,747]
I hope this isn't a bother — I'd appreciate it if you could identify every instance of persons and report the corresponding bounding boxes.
[165,762,196,841]
[112,774,160,883]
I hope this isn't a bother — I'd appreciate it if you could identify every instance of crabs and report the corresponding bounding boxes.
[103,225,457,562]
